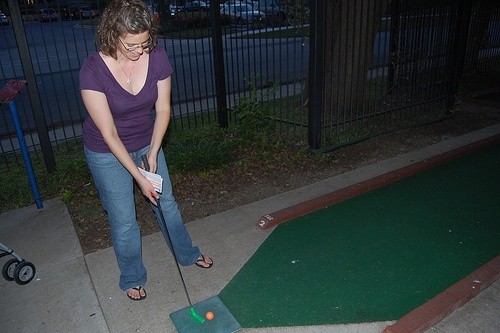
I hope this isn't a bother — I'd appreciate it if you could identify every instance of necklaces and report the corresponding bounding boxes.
[117,59,136,85]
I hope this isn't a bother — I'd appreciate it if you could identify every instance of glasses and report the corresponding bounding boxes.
[117,32,152,53]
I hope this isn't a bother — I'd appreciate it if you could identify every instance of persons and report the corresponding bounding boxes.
[79,0,213,301]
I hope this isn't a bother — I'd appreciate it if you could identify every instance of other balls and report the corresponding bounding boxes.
[205,311,214,320]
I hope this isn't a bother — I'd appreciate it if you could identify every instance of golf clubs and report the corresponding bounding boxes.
[140,153,205,325]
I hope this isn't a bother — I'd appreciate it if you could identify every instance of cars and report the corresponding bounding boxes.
[183,1,210,13]
[76,5,96,20]
[22,7,59,23]
[220,3,267,25]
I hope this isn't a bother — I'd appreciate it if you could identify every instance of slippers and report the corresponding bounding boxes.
[126,284,147,300]
[195,254,213,268]
